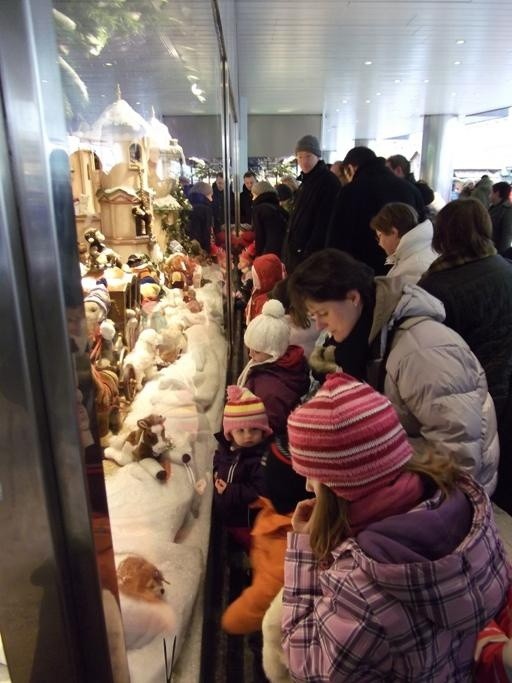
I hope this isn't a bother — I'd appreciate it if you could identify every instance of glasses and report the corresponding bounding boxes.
[374,231,382,243]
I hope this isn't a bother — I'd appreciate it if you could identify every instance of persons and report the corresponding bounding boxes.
[177,175,188,191]
[182,180,218,257]
[203,132,512,682]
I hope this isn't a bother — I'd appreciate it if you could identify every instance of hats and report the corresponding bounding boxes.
[295,134,321,158]
[237,299,292,387]
[222,383,274,444]
[286,366,414,504]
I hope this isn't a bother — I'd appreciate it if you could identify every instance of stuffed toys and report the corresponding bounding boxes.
[66,228,206,682]
[67,227,204,647]
